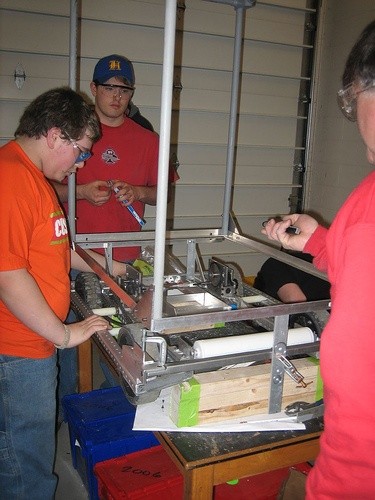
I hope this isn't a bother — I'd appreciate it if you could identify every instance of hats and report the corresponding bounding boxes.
[93,54,135,87]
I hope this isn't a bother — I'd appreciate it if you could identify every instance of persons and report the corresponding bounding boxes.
[253,209,332,337]
[0,86,114,500]
[44,53,180,426]
[259,20,375,500]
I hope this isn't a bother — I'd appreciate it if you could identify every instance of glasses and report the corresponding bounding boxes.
[64,131,93,163]
[336,76,375,122]
[97,83,134,100]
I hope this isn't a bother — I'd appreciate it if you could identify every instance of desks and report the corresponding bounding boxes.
[77,314,323,500]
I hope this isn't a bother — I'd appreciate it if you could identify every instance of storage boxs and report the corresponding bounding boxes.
[62,386,291,500]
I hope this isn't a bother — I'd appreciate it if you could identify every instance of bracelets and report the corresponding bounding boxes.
[54,323,71,349]
